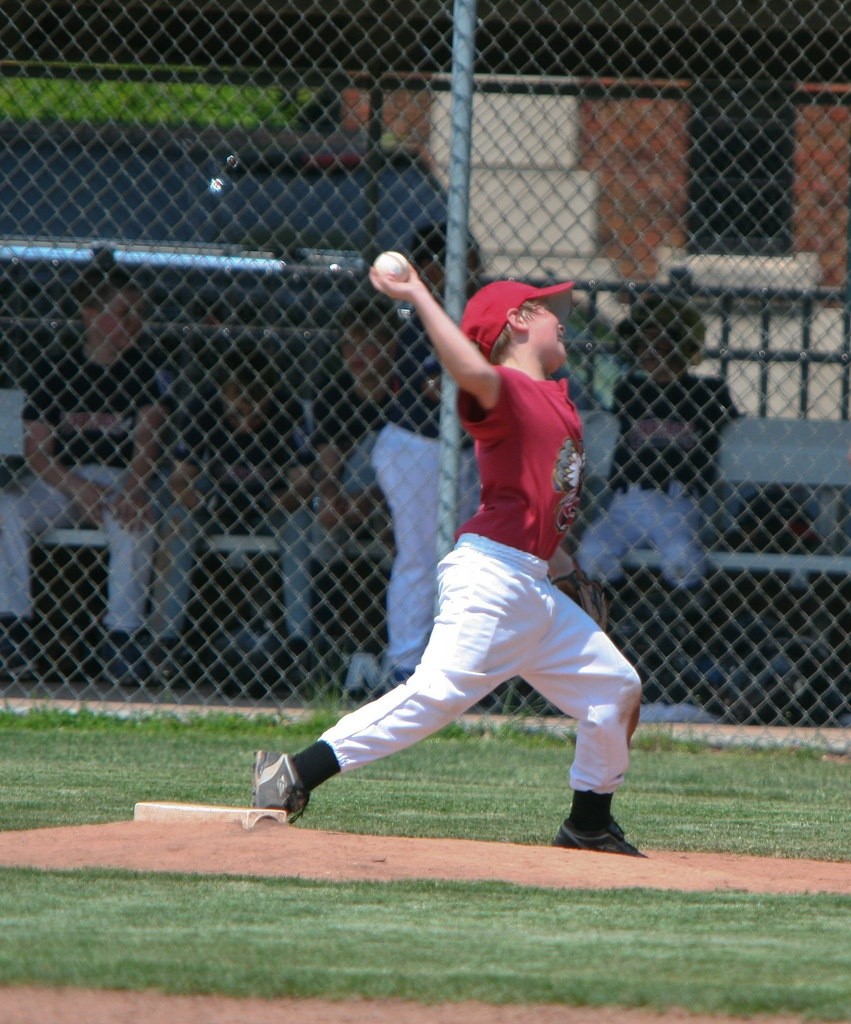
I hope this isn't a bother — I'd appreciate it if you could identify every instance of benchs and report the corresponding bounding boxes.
[0,389,851,573]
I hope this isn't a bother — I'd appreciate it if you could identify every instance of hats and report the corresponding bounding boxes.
[616,286,707,359]
[462,281,575,361]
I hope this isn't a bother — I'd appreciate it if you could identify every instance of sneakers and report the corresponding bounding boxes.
[552,814,648,858]
[252,749,310,824]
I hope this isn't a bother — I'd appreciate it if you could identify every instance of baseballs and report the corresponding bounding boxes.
[373,250,411,284]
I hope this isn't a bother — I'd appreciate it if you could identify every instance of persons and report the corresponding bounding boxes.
[1,230,475,698]
[576,294,730,676]
[255,260,642,854]
